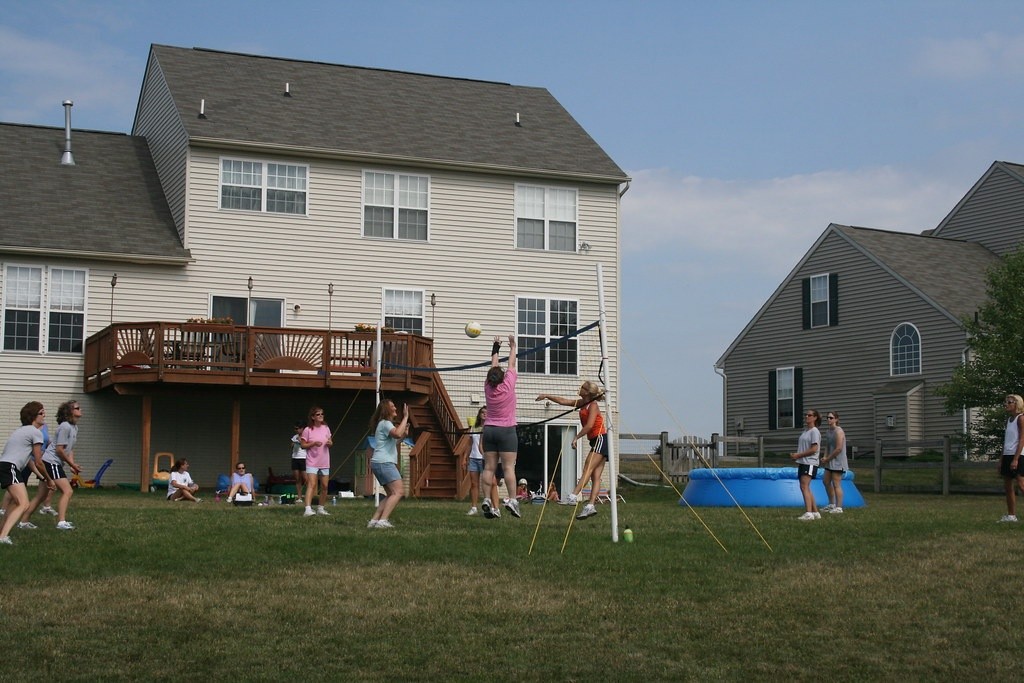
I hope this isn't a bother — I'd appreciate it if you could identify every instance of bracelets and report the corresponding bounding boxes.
[42,478,45,481]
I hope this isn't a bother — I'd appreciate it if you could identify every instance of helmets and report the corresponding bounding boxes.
[518,478,527,485]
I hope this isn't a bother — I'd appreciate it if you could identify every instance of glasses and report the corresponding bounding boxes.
[316,413,324,416]
[38,412,42,416]
[582,385,589,391]
[1007,400,1014,404]
[294,427,298,430]
[804,413,814,417]
[74,406,80,410]
[827,417,833,420]
[238,467,245,470]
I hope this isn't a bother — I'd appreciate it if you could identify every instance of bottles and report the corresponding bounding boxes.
[332,497,336,506]
[624,524,634,543]
[216,490,220,501]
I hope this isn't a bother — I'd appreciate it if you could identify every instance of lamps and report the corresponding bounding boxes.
[248,276,255,325]
[327,282,335,330]
[111,273,117,323]
[431,293,436,338]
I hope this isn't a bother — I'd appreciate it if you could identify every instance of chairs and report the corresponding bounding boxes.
[174,318,206,369]
[206,316,241,371]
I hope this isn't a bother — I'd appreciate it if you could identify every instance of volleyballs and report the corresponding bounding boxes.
[465,321,482,338]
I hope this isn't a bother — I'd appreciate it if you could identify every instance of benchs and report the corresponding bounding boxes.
[264,467,333,491]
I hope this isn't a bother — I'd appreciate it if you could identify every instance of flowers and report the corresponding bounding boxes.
[354,323,397,332]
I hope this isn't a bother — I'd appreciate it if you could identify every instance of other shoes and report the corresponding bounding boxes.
[175,494,331,517]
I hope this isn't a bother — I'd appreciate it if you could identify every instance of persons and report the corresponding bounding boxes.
[167,457,204,502]
[0,401,57,545]
[535,380,609,520]
[518,479,527,495]
[300,407,332,517]
[291,424,306,503]
[820,411,848,514]
[547,482,559,500]
[17,400,84,530]
[0,421,58,516]
[789,409,821,520]
[461,405,500,517]
[482,334,521,519]
[226,463,255,503]
[996,394,1024,524]
[366,398,410,528]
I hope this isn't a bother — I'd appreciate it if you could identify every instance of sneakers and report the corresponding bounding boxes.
[819,503,843,514]
[467,498,521,518]
[39,505,58,517]
[797,511,821,521]
[0,508,6,515]
[0,536,12,545]
[367,519,395,528]
[557,494,577,506]
[55,521,73,530]
[17,521,37,530]
[576,505,597,520]
[996,515,1018,524]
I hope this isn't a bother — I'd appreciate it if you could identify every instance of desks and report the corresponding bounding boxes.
[154,339,222,368]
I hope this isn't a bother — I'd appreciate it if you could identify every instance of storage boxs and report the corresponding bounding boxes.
[338,491,355,498]
